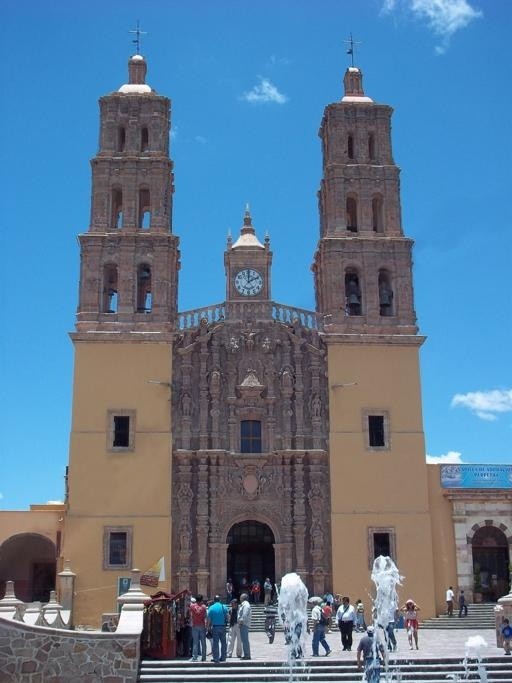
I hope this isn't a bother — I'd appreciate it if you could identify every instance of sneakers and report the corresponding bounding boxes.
[310,654,319,657]
[325,650,333,656]
[191,653,251,662]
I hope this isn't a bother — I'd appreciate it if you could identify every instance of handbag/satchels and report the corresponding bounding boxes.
[338,619,345,628]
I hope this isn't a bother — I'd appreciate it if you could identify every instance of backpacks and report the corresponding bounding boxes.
[315,607,329,626]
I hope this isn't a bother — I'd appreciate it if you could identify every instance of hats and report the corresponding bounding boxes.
[406,599,415,606]
[367,625,374,634]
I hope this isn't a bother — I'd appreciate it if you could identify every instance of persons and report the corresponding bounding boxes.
[378,279,393,316]
[496,568,505,596]
[479,566,490,603]
[149,570,420,683]
[501,619,512,655]
[446,586,454,616]
[459,590,467,618]
[32,568,44,602]
[347,274,361,315]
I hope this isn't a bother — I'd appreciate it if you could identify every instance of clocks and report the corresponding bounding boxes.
[233,266,265,297]
